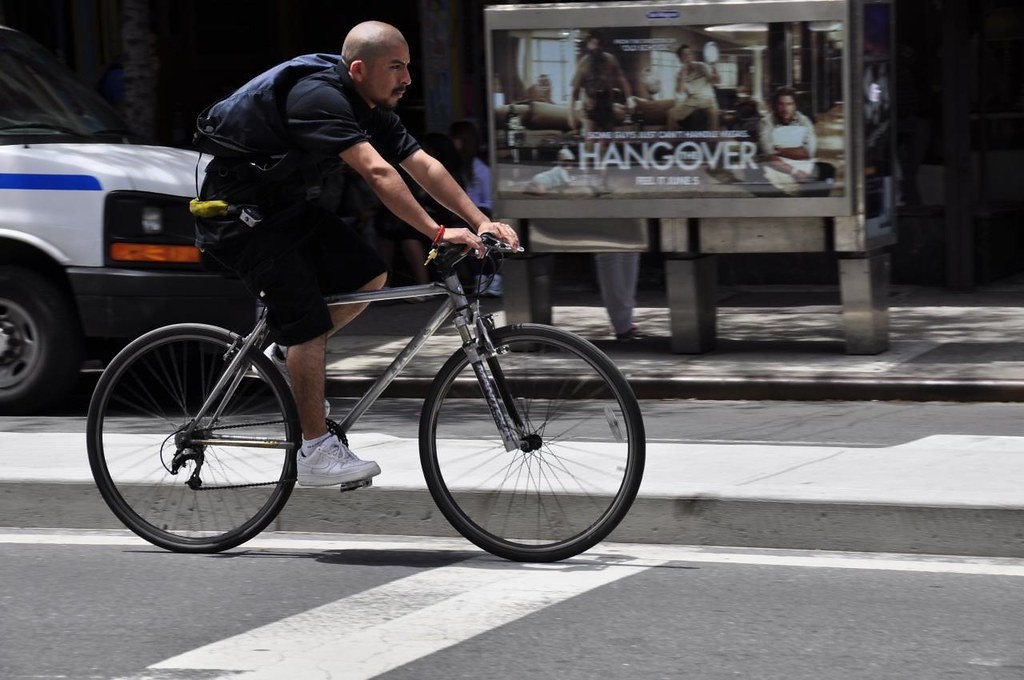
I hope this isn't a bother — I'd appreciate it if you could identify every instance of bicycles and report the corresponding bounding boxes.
[87,232,646,562]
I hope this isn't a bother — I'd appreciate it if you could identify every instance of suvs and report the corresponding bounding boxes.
[0,26,256,416]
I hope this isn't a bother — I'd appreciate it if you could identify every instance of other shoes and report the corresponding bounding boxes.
[476,272,503,296]
[616,326,647,342]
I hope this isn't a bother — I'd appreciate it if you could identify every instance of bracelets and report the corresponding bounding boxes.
[432,224,445,246]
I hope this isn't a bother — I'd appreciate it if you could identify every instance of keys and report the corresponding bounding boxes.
[424,249,438,266]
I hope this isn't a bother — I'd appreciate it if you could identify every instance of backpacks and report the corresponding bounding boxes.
[190,52,342,163]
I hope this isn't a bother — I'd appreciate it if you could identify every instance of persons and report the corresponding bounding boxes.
[667,44,836,198]
[595,253,647,340]
[372,121,504,305]
[567,35,637,197]
[522,148,576,196]
[195,20,520,487]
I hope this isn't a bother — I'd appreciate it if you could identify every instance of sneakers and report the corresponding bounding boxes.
[296,435,380,487]
[251,346,332,420]
